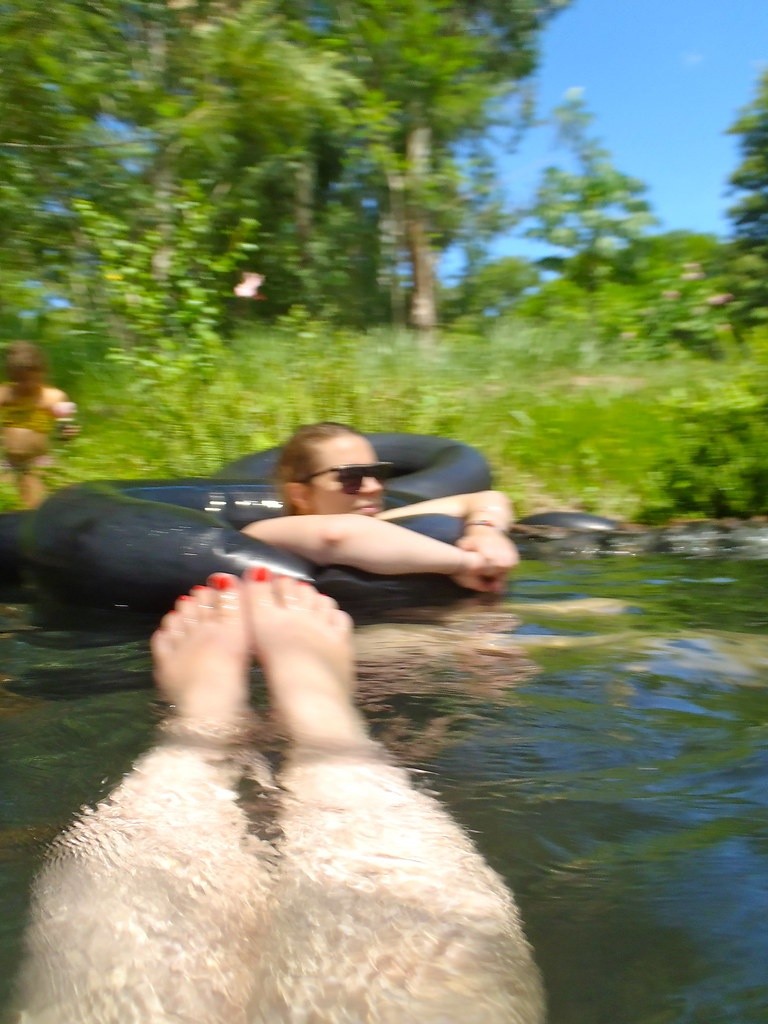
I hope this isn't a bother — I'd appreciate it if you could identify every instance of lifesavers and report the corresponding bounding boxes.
[32,477,475,613]
[213,427,492,500]
[517,510,620,530]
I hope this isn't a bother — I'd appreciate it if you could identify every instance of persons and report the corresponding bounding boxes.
[239,422,521,592]
[148,563,366,738]
[0,342,81,509]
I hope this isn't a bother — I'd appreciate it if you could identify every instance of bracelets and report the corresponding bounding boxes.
[462,520,504,534]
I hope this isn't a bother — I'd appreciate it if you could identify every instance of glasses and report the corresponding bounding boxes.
[295,463,397,492]
